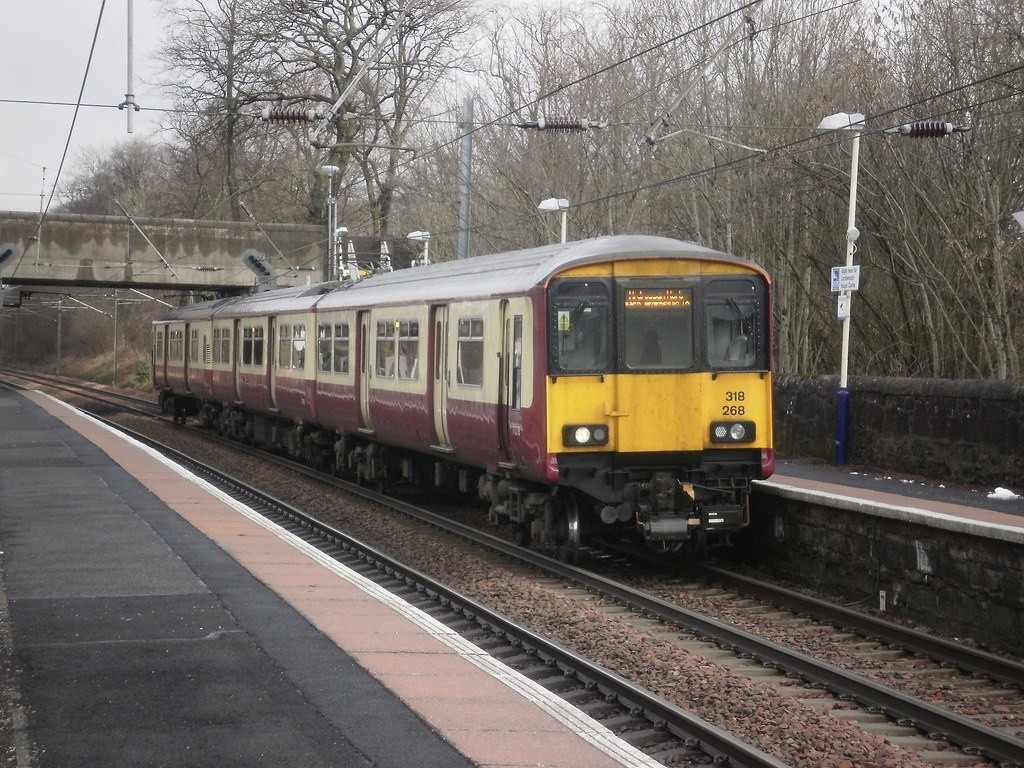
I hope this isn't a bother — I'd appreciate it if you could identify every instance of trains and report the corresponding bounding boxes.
[149,233,777,562]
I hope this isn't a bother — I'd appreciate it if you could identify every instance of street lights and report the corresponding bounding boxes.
[321,165,339,281]
[539,196,569,244]
[407,232,430,263]
[333,224,348,281]
[818,114,867,467]
